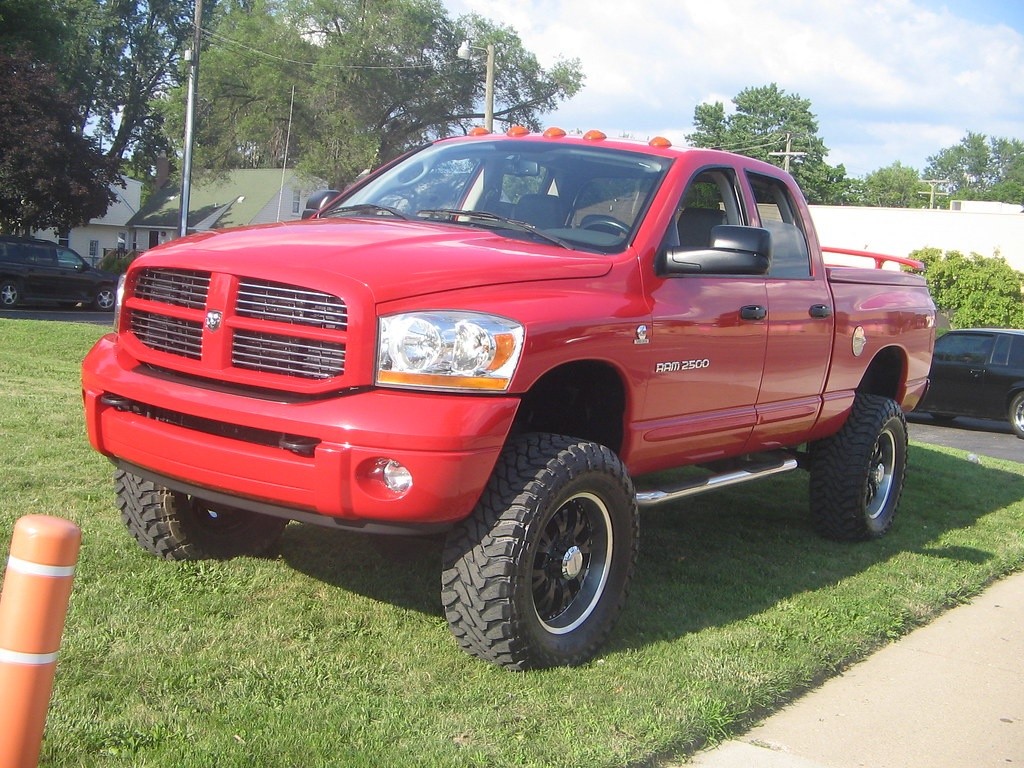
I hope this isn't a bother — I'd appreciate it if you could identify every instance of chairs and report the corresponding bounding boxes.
[513,191,571,232]
[674,206,728,247]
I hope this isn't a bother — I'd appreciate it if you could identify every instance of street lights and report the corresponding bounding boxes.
[457,40,494,134]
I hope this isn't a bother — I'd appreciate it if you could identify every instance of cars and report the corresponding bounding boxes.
[1,235,120,312]
[908,328,1024,436]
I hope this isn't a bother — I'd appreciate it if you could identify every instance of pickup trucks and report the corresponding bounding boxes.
[87,130,934,666]
